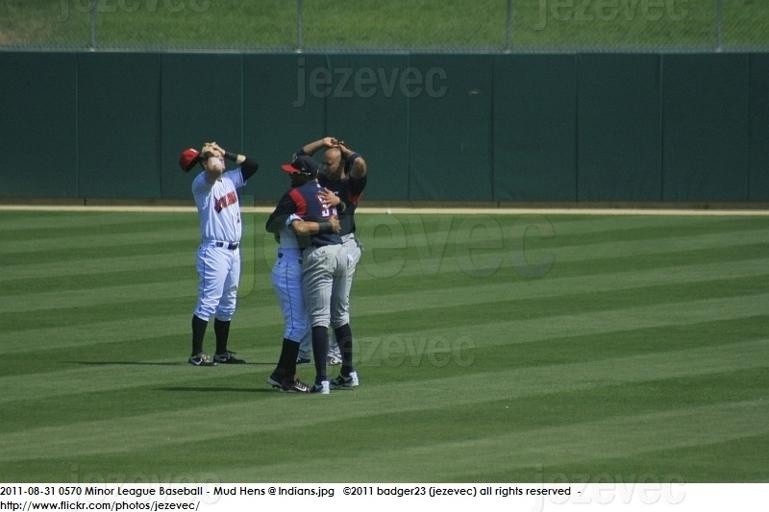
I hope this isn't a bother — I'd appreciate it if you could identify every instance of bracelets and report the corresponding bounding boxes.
[225,150,237,162]
[203,151,214,160]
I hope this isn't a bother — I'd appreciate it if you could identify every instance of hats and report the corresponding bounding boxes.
[280,155,320,178]
[178,148,201,173]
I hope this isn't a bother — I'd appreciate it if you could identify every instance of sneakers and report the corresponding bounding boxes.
[266,369,359,394]
[296,356,311,364]
[326,343,343,366]
[188,350,247,367]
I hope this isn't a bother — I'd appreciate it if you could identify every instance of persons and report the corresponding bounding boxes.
[178,141,258,366]
[266,137,367,395]
